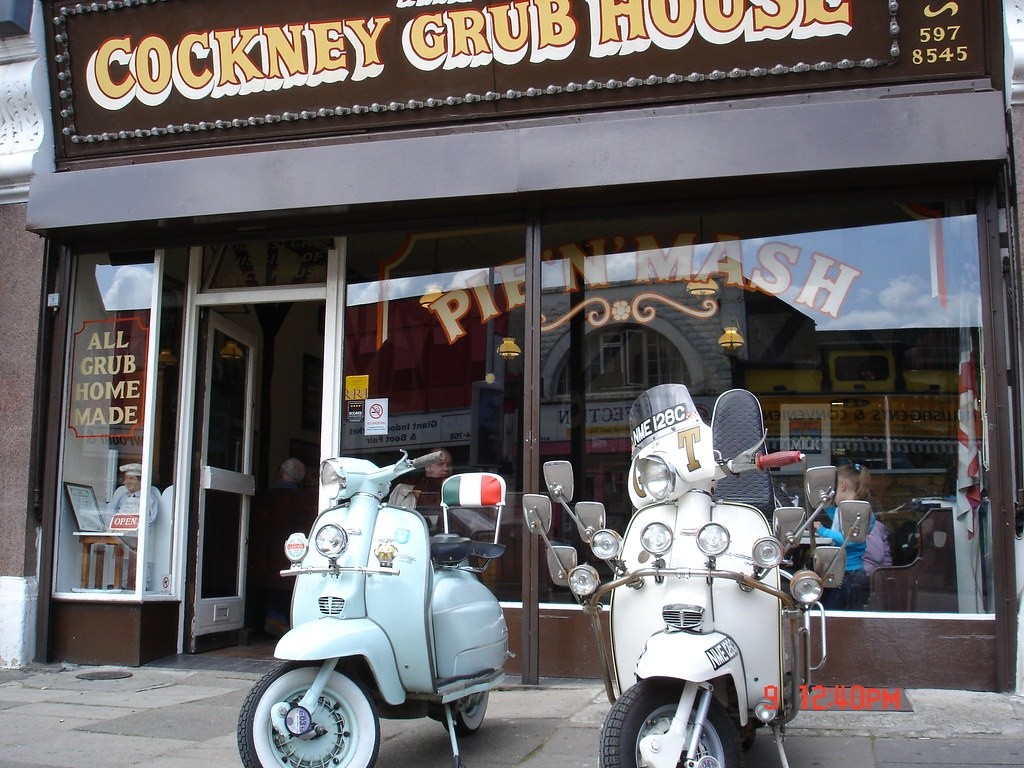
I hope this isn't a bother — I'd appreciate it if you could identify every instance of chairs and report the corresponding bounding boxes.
[712,388,776,522]
[429,472,507,572]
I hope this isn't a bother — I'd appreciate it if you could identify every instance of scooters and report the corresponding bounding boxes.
[521,380,872,768]
[236,449,518,768]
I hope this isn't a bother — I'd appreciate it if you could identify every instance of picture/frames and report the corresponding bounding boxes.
[778,403,833,470]
[63,481,107,532]
[288,438,320,467]
[301,352,324,430]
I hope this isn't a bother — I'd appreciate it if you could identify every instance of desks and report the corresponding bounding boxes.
[71,532,138,590]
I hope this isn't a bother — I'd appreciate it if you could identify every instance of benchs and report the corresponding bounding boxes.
[867,507,959,614]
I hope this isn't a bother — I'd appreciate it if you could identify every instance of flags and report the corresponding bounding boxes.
[955,298,985,541]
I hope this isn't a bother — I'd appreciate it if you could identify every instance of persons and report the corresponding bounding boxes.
[424,447,454,478]
[244,456,305,638]
[106,463,159,590]
[811,462,893,611]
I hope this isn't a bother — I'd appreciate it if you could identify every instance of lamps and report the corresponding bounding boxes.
[718,316,745,351]
[220,341,242,359]
[497,338,521,357]
[687,278,719,295]
[158,349,178,365]
